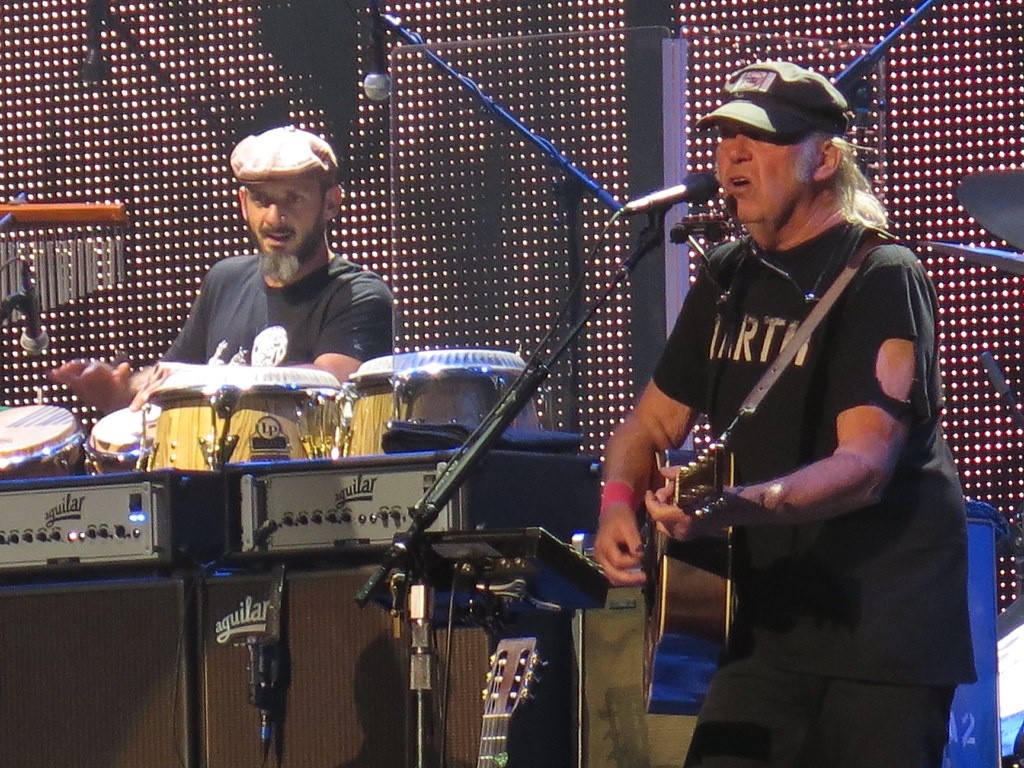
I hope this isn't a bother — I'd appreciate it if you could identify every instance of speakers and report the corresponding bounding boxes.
[0,565,697,768]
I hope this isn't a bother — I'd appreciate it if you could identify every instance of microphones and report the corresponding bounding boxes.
[257,633,274,758]
[618,172,719,215]
[361,1,391,100]
[20,260,49,355]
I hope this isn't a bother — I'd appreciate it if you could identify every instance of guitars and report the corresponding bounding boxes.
[644,447,738,717]
[474,636,547,768]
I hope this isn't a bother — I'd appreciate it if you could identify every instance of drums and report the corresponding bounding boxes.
[146,367,343,472]
[333,349,542,458]
[82,404,162,475]
[0,405,87,480]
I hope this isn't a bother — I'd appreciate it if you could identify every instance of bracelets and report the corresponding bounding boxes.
[600,481,637,511]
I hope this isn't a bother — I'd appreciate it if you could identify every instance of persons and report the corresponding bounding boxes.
[592,62,979,768]
[45,125,395,412]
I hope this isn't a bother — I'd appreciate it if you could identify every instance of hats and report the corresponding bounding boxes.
[696,62,848,135]
[230,124,338,185]
